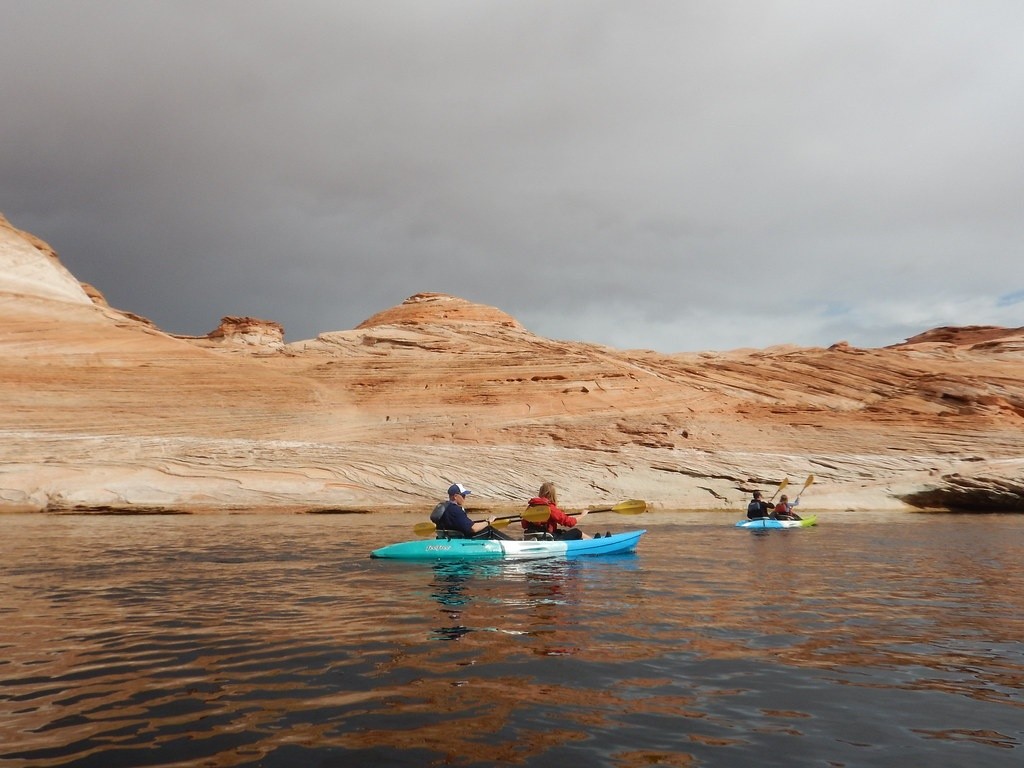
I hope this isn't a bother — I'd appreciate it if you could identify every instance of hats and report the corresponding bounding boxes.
[448,483,471,494]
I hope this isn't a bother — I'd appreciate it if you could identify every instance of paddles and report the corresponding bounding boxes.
[766,477,789,504]
[794,475,814,502]
[412,500,646,536]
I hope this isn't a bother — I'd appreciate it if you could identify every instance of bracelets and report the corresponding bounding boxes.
[486,520,490,526]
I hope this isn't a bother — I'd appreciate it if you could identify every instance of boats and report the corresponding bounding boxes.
[734,514,818,528]
[369,529,647,560]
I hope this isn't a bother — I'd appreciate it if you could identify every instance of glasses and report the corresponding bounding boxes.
[457,493,466,498]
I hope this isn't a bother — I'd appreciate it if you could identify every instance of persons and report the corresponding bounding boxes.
[748,492,778,520]
[520,482,612,541]
[436,483,538,541]
[775,495,803,520]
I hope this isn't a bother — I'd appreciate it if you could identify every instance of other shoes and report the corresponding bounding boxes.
[530,537,537,541]
[594,533,601,538]
[605,531,611,538]
